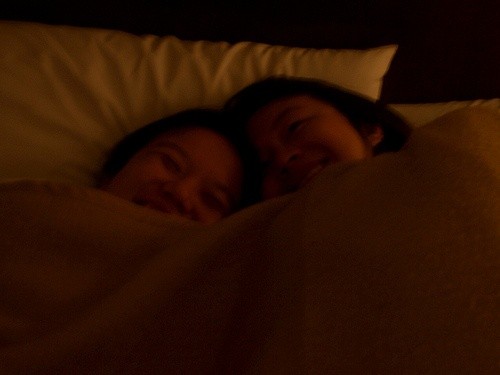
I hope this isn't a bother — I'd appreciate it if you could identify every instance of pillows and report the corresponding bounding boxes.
[0,21,398,185]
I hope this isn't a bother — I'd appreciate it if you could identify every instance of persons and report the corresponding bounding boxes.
[223,75,415,195]
[91,105,258,228]
